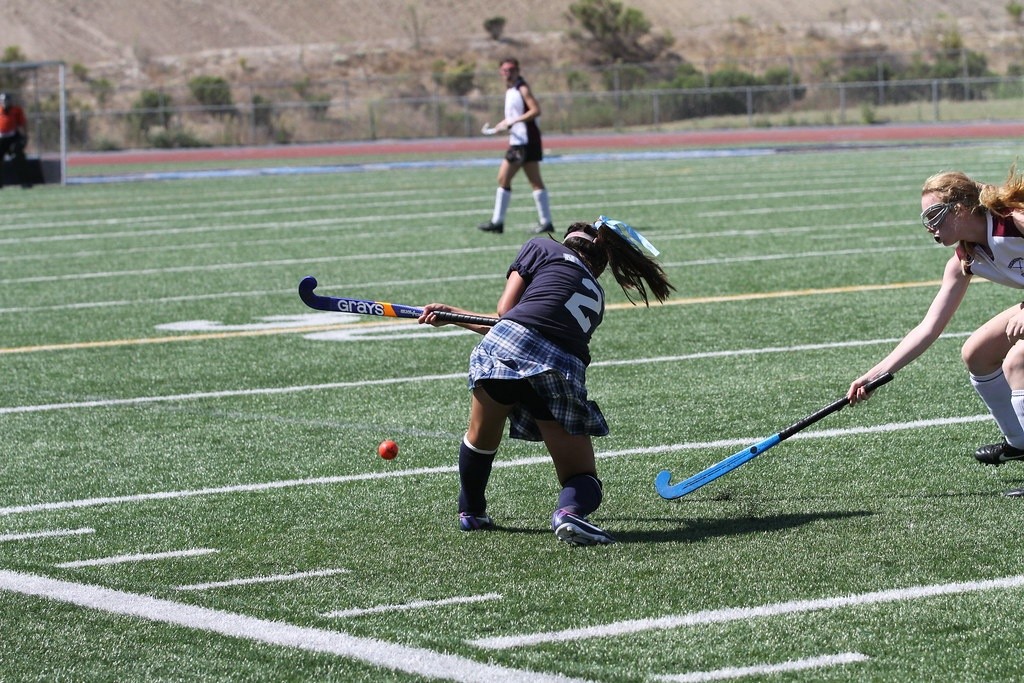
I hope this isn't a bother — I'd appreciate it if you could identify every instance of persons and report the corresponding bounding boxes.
[846,169,1024,498]
[419,214,678,547]
[0,93,33,188]
[479,57,555,235]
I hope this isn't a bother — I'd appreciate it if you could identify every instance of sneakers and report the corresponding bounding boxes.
[552,509,616,547]
[479,221,504,234]
[529,223,555,235]
[975,442,1024,467]
[459,512,490,531]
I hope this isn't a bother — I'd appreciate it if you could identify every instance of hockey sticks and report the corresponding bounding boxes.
[298,275,499,326]
[481,123,512,135]
[654,371,894,501]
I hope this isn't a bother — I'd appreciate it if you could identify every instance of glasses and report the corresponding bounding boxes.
[920,202,958,233]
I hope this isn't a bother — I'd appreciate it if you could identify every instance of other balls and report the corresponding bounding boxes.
[379,440,399,460]
